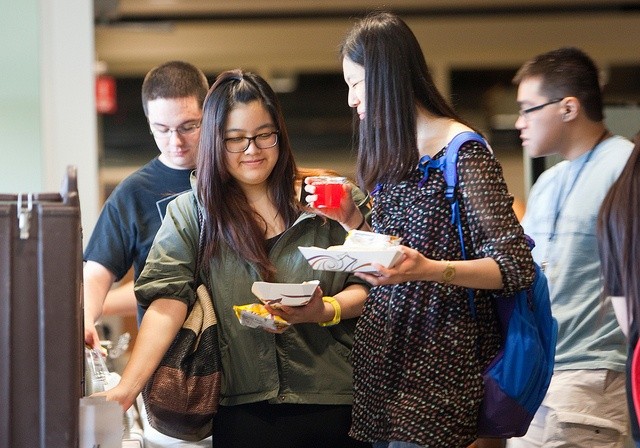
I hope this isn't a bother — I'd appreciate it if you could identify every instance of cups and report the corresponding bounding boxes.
[78,399,124,448]
[311,174,348,212]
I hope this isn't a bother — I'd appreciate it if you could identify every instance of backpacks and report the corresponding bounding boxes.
[369,132,558,439]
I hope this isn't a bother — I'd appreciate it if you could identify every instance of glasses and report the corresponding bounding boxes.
[224,130,279,153]
[518,100,561,115]
[151,118,202,136]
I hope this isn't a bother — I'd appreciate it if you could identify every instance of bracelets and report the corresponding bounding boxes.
[356,214,365,230]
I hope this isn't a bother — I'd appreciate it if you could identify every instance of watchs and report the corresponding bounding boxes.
[438,260,456,284]
[318,296,341,327]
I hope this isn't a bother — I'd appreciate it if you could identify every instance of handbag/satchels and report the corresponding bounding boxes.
[142,284,221,441]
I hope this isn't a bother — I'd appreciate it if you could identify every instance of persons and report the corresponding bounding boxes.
[596,129,640,448]
[506,47,638,448]
[89,69,371,448]
[304,13,535,448]
[83,60,210,350]
